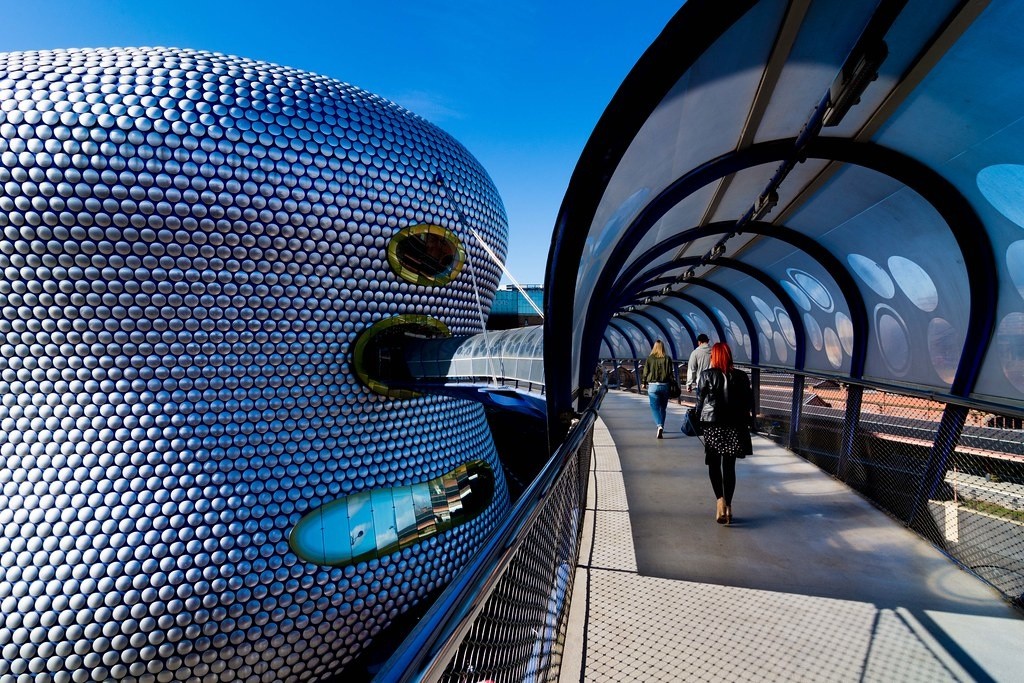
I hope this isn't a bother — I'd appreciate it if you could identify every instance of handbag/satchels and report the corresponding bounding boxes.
[680,408,704,436]
[667,378,681,398]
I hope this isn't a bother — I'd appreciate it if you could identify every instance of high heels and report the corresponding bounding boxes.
[716,497,727,524]
[725,506,732,524]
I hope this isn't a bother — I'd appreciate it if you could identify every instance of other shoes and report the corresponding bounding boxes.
[657,425,664,439]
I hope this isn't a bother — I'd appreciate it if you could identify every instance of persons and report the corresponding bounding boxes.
[695,343,753,523]
[687,334,712,409]
[642,339,673,439]
